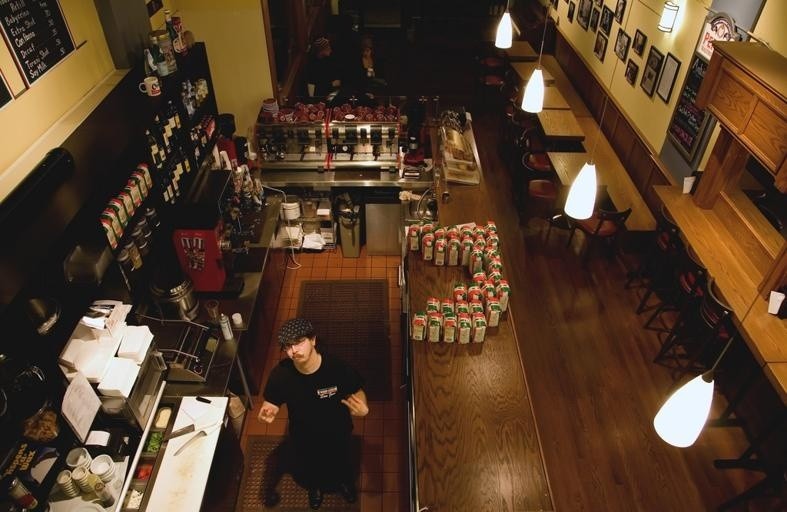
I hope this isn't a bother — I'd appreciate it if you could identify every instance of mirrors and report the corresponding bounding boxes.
[718,154,787,264]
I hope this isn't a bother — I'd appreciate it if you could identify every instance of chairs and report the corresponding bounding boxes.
[473,41,633,268]
[622,201,734,396]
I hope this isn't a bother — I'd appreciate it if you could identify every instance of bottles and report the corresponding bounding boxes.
[163,8,181,58]
[219,313,233,340]
[231,162,242,191]
[192,239,199,270]
[244,169,252,189]
[93,482,115,508]
[143,48,163,87]
[241,176,252,206]
[198,240,205,272]
[2,474,38,510]
[189,126,208,160]
[182,239,190,267]
[181,80,201,117]
[162,146,191,202]
[188,239,194,268]
[151,36,168,77]
[145,101,182,168]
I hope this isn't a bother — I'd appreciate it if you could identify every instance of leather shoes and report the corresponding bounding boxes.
[308,484,321,510]
[338,479,356,503]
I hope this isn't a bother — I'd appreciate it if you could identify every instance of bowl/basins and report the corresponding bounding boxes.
[56,470,80,497]
[91,454,116,482]
[65,448,92,470]
[72,466,94,493]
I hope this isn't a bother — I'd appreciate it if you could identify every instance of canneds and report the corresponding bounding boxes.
[116,249,137,276]
[135,216,156,246]
[143,205,163,233]
[127,227,150,254]
[120,238,144,271]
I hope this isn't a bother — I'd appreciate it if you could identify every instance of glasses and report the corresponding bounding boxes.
[281,338,305,352]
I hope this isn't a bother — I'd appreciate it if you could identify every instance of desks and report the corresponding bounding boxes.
[712,362,787,512]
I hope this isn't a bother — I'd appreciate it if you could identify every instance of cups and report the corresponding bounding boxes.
[138,77,161,97]
[232,313,242,325]
[204,299,219,318]
[442,191,453,205]
[220,150,230,170]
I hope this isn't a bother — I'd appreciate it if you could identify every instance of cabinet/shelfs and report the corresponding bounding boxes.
[66,138,170,301]
[695,38,787,194]
[108,40,222,207]
[252,105,329,172]
[325,108,403,172]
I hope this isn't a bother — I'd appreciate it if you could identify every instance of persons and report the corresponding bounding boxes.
[549,0,657,81]
[302,36,342,106]
[357,30,393,94]
[258,317,369,510]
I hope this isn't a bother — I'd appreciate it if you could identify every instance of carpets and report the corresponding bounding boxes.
[295,278,395,406]
[232,434,362,512]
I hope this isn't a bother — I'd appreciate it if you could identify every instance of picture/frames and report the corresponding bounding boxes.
[548,0,682,104]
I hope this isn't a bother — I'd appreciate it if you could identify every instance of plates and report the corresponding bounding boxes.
[279,201,301,220]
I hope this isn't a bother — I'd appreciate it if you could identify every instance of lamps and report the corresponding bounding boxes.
[657,1,680,35]
[652,250,787,449]
[494,0,513,50]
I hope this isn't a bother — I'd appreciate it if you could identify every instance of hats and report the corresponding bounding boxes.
[277,319,313,347]
